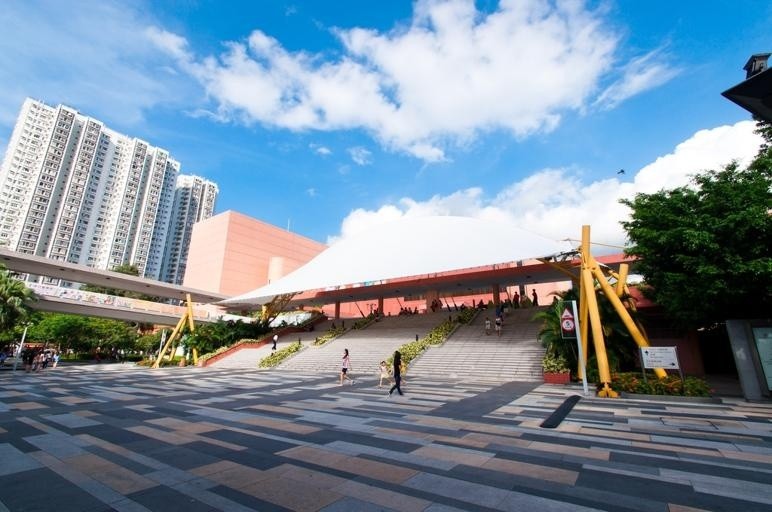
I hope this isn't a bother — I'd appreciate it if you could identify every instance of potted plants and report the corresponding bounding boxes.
[540,355,572,387]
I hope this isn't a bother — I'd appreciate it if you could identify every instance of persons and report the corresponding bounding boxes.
[0,352,8,368]
[21,347,60,374]
[550,295,558,309]
[337,348,355,387]
[395,298,443,320]
[271,331,280,350]
[387,350,405,396]
[531,288,539,307]
[459,303,466,312]
[328,319,335,332]
[378,361,393,389]
[483,291,521,338]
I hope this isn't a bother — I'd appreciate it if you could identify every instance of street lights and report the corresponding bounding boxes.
[12,321,35,373]
